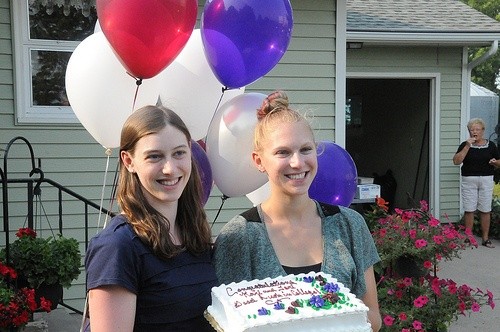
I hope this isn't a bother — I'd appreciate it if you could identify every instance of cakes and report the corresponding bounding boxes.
[207,271,372,332]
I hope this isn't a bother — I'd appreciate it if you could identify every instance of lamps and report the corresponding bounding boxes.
[346,42,364,49]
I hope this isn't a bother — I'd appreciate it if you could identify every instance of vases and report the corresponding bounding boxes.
[395,255,429,280]
[37,283,63,311]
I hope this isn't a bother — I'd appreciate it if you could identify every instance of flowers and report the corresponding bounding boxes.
[364,195,496,332]
[0,226,82,332]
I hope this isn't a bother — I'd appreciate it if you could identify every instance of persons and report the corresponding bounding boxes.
[86,106,218,332]
[212,91,381,331]
[453,119,500,249]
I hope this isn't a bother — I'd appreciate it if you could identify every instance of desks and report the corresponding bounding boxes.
[351,199,375,211]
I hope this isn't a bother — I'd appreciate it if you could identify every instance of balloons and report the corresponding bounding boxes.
[246,181,272,207]
[189,139,213,204]
[206,93,270,197]
[308,140,358,207]
[95,0,198,80]
[200,0,293,89]
[65,33,161,157]
[152,28,246,142]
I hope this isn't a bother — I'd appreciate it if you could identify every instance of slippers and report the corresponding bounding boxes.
[482,240,496,248]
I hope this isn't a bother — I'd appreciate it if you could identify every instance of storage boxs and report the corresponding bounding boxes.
[354,177,380,200]
